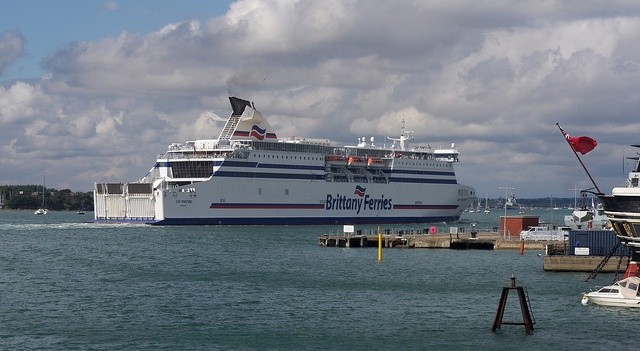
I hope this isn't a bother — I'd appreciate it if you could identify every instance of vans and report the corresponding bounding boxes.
[518,225,546,238]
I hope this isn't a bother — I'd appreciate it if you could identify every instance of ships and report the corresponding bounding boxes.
[93,96,476,226]
[556,122,639,280]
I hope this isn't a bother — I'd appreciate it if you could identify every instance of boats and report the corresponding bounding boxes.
[584,276,639,306]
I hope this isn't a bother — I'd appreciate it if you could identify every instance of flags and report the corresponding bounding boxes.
[560,128,597,155]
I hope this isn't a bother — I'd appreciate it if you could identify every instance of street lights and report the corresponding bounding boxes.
[567,188,583,208]
[498,186,515,240]
